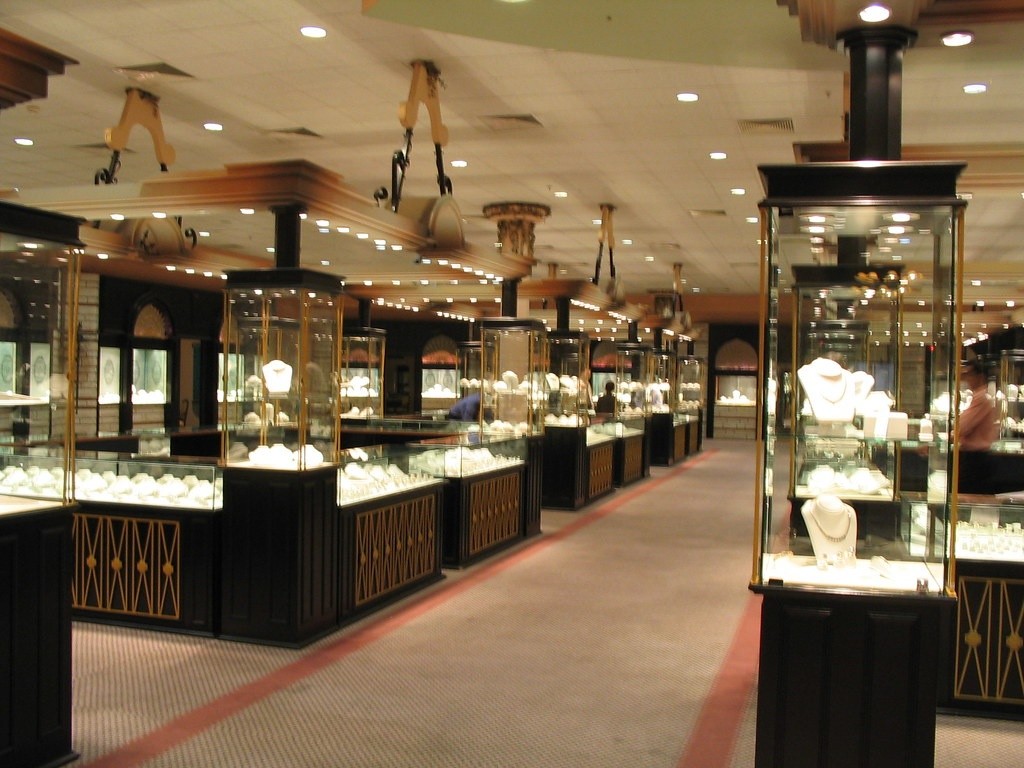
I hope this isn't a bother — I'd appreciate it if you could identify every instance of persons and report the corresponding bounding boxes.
[954,357,1000,495]
[449,393,493,442]
[579,369,594,409]
[597,382,619,415]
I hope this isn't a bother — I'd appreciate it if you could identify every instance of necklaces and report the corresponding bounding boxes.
[808,508,852,541]
[821,379,848,403]
